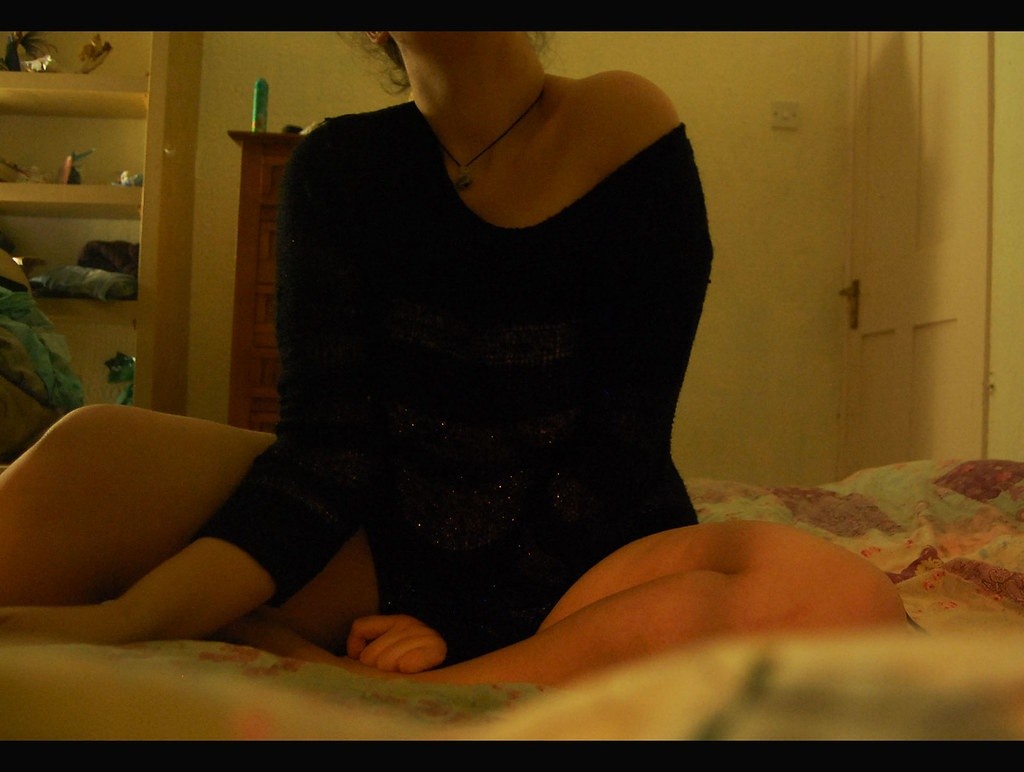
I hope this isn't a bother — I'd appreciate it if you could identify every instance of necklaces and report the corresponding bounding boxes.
[411,82,548,192]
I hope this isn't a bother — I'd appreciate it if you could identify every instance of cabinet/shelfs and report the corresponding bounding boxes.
[229,128,298,430]
[0,30,164,411]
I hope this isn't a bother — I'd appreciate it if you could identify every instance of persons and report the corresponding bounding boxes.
[0,30,907,685]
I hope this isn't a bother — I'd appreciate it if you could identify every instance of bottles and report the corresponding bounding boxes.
[250,76,269,131]
